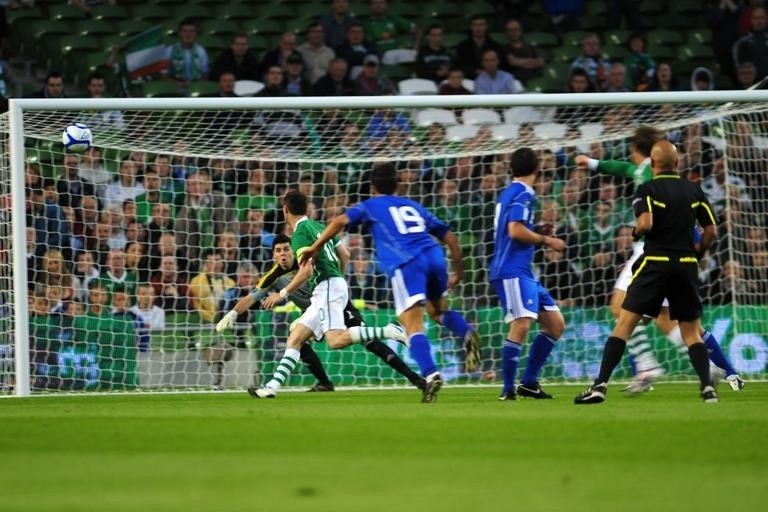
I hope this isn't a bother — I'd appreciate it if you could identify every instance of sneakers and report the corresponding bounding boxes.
[700,384,718,403]
[726,374,745,391]
[386,319,409,348]
[248,384,278,399]
[497,393,517,400]
[516,382,556,400]
[573,380,609,403]
[618,366,668,393]
[463,329,483,373]
[420,371,443,404]
[306,381,335,392]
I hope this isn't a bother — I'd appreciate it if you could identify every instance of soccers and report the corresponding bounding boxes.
[63,123,91,153]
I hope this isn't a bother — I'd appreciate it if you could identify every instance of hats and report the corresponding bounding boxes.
[364,54,380,65]
[286,53,303,64]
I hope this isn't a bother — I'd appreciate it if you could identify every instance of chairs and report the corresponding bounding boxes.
[2,1,768,349]
